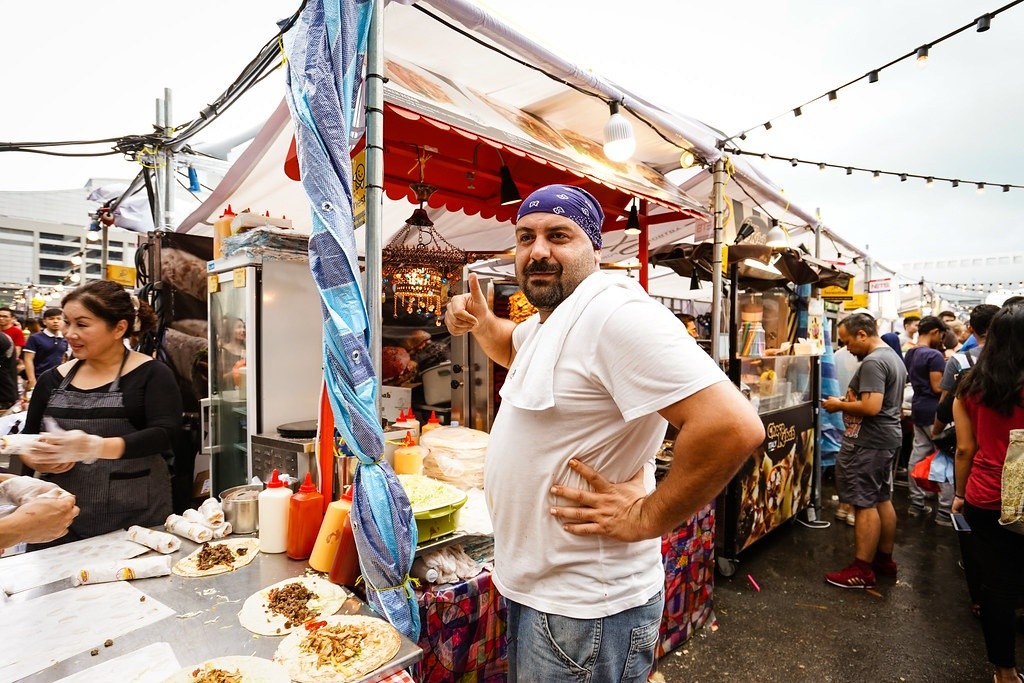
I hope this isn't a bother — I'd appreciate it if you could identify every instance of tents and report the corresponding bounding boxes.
[85,1,896,638]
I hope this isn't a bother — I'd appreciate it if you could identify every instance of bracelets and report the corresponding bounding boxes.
[955,495,964,499]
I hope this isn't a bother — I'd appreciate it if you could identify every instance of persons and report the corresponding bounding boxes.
[19,280,182,553]
[444,184,766,683]
[821,314,907,588]
[0,308,74,411]
[217,318,246,390]
[0,473,80,556]
[834,296,1024,683]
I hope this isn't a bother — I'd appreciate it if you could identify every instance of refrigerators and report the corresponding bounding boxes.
[198,250,323,503]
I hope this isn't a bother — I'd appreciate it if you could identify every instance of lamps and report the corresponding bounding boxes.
[467,141,522,206]
[820,164,826,170]
[977,184,985,191]
[601,102,635,163]
[734,149,742,155]
[1003,186,1009,192]
[901,174,907,181]
[734,223,755,246]
[952,181,959,188]
[927,178,934,185]
[765,219,786,247]
[873,172,880,179]
[382,150,469,326]
[791,159,797,167]
[690,267,703,290]
[679,149,706,168]
[740,17,991,141]
[763,154,769,160]
[846,168,853,175]
[624,197,641,235]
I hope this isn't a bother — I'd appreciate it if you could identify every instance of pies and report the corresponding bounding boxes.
[238,576,348,636]
[270,615,401,683]
[418,425,491,491]
[158,655,293,683]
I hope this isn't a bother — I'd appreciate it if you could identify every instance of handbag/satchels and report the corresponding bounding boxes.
[999,429,1024,534]
[19,369,29,380]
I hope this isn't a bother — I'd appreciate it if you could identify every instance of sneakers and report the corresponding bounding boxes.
[825,560,898,589]
[935,512,953,526]
[908,503,932,517]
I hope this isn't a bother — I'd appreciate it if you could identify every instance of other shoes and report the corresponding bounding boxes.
[835,509,856,526]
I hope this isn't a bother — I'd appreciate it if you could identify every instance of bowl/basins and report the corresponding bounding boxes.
[218,485,264,535]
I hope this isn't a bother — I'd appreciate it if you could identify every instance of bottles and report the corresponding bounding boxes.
[405,407,420,445]
[421,410,441,436]
[390,409,413,428]
[393,431,421,475]
[328,510,358,586]
[737,321,766,358]
[308,483,353,573]
[287,472,324,561]
[258,468,293,554]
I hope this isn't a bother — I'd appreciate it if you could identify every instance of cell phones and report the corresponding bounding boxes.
[950,513,972,532]
[819,398,827,402]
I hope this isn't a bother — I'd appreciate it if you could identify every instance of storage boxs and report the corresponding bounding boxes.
[382,386,412,423]
[230,213,291,235]
